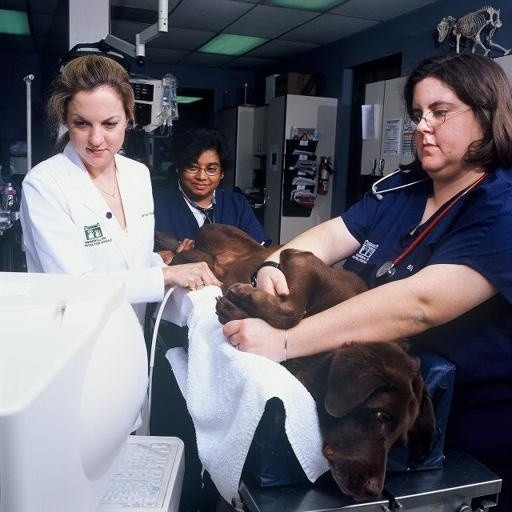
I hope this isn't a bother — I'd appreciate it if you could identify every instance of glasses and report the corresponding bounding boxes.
[180,164,221,175]
[411,107,473,127]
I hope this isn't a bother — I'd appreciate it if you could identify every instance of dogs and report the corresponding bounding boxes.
[153,223,436,501]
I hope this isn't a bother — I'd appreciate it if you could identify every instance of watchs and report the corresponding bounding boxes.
[251,261,281,288]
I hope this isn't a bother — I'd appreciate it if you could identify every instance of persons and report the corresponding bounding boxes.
[154,127,272,265]
[223,51,512,512]
[19,54,223,435]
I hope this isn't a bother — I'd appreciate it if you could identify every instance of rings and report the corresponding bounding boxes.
[235,346,239,351]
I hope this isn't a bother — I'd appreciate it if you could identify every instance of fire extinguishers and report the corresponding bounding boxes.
[317,156,333,195]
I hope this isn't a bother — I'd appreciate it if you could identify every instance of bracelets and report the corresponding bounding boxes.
[283,329,288,362]
